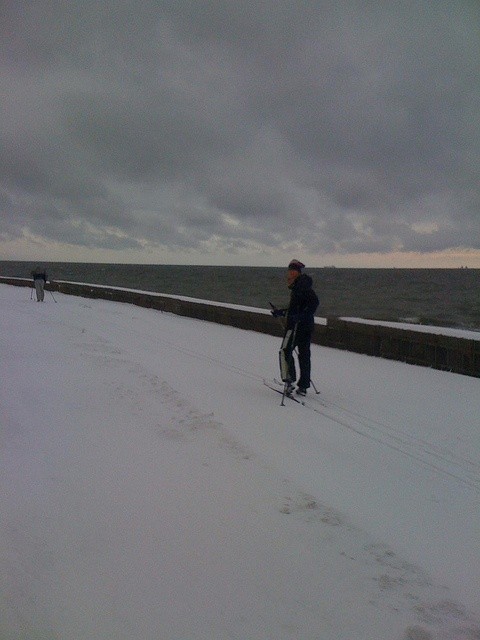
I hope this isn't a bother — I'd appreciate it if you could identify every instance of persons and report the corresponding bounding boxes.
[273,259,319,396]
[32,265,48,302]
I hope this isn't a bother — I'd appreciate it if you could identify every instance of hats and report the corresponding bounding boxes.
[288,259,304,273]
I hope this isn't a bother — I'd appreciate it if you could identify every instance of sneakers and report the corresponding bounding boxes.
[286,384,295,394]
[296,388,306,396]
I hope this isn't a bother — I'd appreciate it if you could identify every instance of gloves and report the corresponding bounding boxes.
[271,308,286,318]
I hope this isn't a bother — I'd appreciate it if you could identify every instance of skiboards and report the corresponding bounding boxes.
[262,377,308,405]
